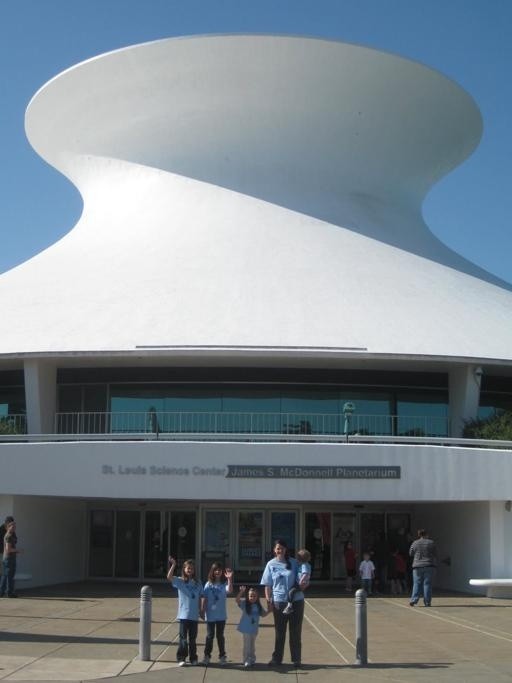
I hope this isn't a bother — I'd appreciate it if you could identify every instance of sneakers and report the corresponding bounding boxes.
[282,605,296,615]
[179,655,226,667]
[244,660,255,667]
[293,660,302,667]
[268,659,282,667]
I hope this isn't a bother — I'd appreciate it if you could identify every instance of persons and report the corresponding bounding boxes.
[200,560,234,666]
[167,555,204,666]
[344,527,413,594]
[236,584,272,667]
[0,521,25,598]
[281,549,312,615]
[0,517,17,585]
[258,538,305,667]
[408,528,438,606]
